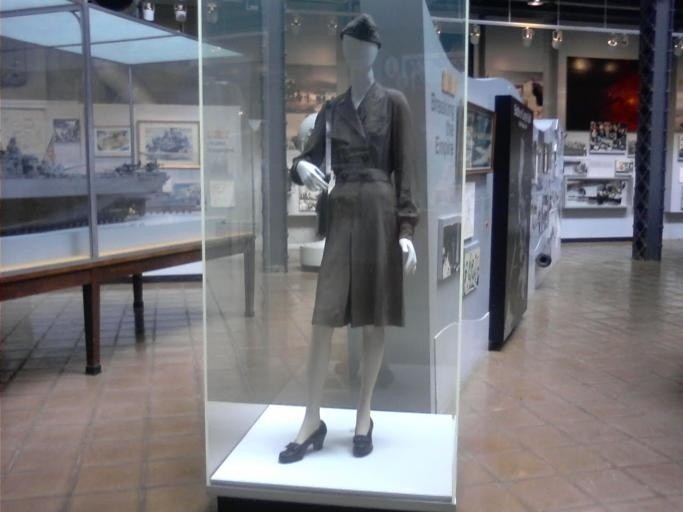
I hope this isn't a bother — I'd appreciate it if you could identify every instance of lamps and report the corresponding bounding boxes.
[468,0,620,50]
[141,1,220,27]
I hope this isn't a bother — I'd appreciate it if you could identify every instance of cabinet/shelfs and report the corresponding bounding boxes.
[563,175,631,212]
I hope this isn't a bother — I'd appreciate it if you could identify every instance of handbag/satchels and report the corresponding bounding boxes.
[315,189,330,240]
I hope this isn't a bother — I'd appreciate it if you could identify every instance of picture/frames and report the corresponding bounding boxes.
[437,213,481,300]
[93,118,200,170]
[455,99,497,177]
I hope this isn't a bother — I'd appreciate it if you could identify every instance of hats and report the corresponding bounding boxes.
[340,13,383,50]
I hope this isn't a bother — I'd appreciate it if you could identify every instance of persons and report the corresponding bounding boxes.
[279,13,421,463]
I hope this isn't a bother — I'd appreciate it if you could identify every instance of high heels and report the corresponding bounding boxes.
[279,418,328,464]
[353,417,374,458]
